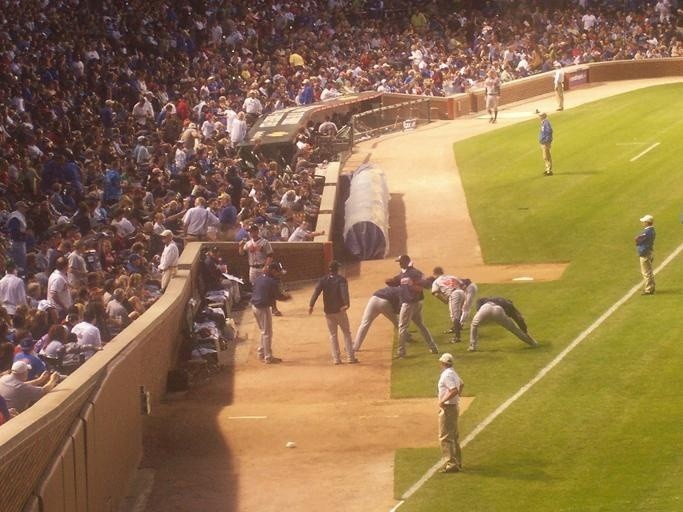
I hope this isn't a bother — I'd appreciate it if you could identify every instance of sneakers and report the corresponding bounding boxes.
[450,337,462,343]
[271,308,280,316]
[428,347,438,355]
[466,345,476,352]
[347,359,360,364]
[393,353,405,360]
[257,352,281,364]
[528,340,539,348]
[334,360,344,365]
[438,466,462,473]
[448,324,464,334]
[640,288,655,295]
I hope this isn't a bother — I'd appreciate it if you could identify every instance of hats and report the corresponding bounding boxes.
[246,225,259,232]
[328,261,345,270]
[11,360,32,375]
[267,261,288,274]
[639,214,654,224]
[158,230,173,239]
[393,254,411,265]
[20,336,37,348]
[438,351,454,366]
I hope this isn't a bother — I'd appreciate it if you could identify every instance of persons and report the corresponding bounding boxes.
[437,352,464,476]
